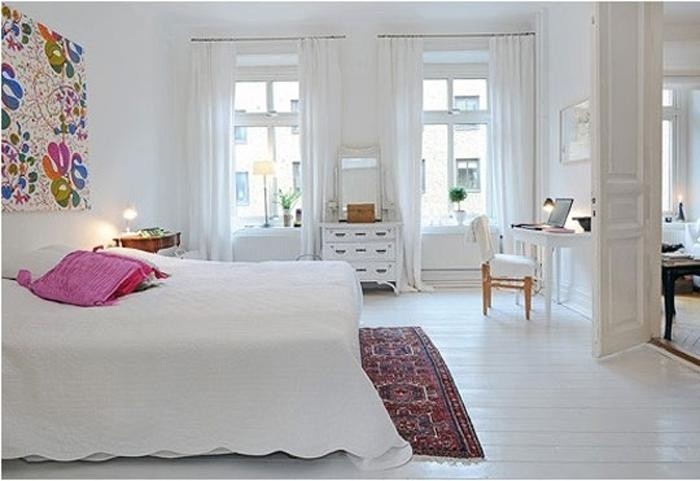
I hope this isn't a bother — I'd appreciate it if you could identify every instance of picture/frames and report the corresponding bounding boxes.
[558,97,591,165]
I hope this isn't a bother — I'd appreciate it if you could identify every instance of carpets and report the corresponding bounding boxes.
[359,326,486,458]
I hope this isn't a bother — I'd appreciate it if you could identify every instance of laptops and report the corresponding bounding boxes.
[522,199,573,230]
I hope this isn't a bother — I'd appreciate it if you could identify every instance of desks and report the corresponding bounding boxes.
[113,230,181,254]
[662,256,700,342]
[510,226,593,313]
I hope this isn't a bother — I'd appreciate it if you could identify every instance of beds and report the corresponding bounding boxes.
[2,247,412,468]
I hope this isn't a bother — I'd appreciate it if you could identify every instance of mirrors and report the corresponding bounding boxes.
[337,144,384,222]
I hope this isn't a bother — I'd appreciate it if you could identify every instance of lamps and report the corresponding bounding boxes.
[120,196,137,232]
[253,144,278,228]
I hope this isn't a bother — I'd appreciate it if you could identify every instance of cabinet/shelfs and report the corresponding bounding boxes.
[317,222,403,297]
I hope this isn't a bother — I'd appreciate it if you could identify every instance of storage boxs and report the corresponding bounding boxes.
[346,203,375,222]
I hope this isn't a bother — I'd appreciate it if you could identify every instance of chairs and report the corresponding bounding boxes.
[472,216,536,320]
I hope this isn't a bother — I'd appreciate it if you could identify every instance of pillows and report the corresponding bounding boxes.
[2,244,85,282]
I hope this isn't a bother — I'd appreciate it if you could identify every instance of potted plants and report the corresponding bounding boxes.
[448,183,467,225]
[274,187,303,227]
